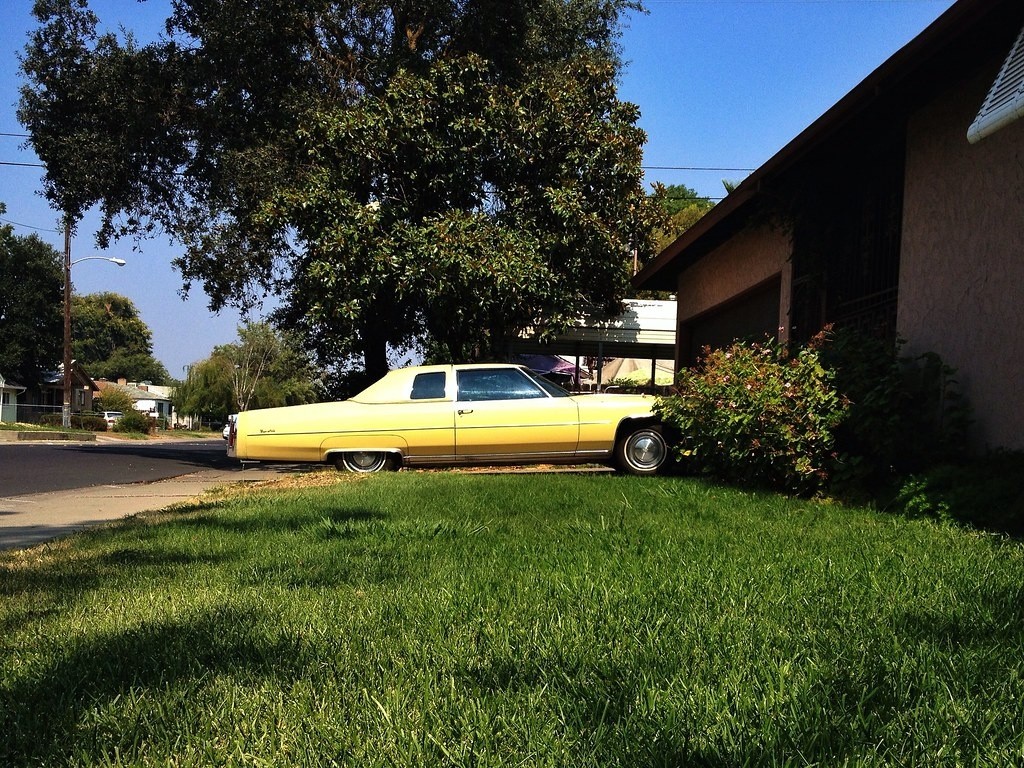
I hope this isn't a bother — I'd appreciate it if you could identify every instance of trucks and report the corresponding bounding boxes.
[223,414,237,439]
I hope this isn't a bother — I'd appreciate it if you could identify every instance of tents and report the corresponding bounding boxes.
[519,353,594,384]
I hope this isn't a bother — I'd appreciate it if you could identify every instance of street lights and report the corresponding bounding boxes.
[63,257,126,429]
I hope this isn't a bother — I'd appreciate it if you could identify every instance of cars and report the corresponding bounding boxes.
[99,412,124,429]
[228,363,670,476]
[201,415,221,431]
